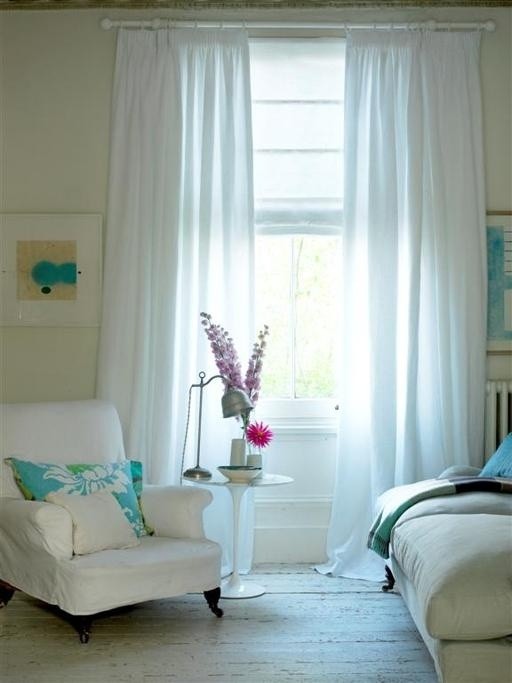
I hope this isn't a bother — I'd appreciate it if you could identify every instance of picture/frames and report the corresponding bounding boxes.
[486,209,512,356]
[0,211,103,327]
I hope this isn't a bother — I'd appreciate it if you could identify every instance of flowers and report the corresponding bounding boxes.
[241,324,272,453]
[198,312,252,452]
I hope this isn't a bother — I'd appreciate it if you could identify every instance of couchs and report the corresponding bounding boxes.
[0,398,225,642]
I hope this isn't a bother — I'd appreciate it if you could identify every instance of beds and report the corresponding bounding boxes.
[367,462,512,683]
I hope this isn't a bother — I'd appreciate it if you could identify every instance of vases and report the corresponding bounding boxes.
[246,452,264,478]
[230,438,248,466]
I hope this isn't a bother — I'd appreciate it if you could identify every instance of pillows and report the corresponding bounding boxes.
[477,432,512,477]
[46,487,141,556]
[4,454,154,557]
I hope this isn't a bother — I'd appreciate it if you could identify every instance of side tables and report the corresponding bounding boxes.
[185,469,295,597]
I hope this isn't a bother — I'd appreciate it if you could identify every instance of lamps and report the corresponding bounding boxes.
[179,370,255,477]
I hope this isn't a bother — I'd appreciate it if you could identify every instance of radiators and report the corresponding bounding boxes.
[484,378,512,467]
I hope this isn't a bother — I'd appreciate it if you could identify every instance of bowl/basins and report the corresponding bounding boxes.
[219,466,261,483]
